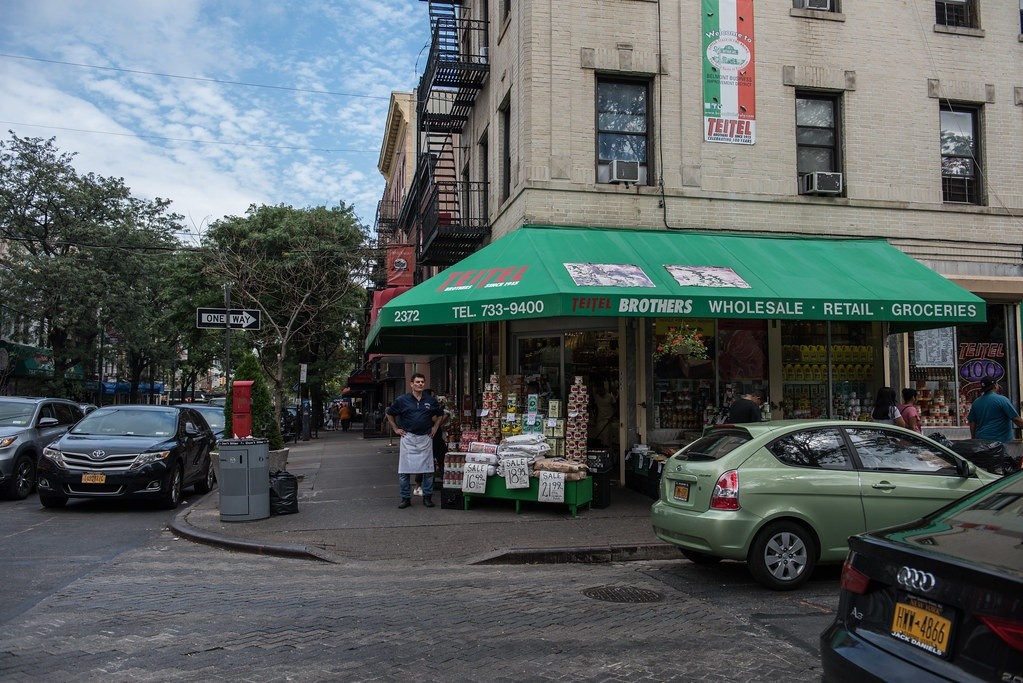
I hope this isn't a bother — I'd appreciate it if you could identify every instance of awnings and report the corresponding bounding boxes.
[340,363,374,399]
[365,226,989,358]
[13,343,94,382]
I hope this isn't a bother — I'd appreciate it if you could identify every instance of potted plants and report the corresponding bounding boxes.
[209,354,290,488]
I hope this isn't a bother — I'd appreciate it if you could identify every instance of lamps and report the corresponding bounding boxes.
[609,160,640,184]
[802,171,844,195]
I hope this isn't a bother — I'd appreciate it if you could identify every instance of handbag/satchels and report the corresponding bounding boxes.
[327,419,334,429]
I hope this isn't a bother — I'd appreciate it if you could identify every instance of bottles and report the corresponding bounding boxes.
[781,320,874,382]
[443,420,480,452]
[443,455,466,504]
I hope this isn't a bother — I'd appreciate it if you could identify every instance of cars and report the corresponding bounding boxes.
[649,418,1008,591]
[818,469,1023,683]
[271,405,301,442]
[185,403,226,441]
[36,404,218,511]
[80,403,97,416]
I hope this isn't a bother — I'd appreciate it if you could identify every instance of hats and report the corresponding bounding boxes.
[980,375,997,393]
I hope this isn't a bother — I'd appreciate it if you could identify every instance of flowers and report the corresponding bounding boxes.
[651,326,712,370]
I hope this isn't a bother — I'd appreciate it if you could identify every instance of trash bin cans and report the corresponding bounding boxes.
[218,438,271,521]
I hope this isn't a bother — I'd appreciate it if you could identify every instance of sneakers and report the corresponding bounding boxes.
[413,486,424,496]
[397,499,411,508]
[422,498,435,507]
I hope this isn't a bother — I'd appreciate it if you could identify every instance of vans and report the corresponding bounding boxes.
[207,398,227,407]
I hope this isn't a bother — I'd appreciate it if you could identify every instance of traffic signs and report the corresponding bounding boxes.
[196,308,260,331]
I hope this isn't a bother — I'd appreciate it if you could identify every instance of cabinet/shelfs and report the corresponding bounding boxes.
[462,475,593,517]
[781,323,884,422]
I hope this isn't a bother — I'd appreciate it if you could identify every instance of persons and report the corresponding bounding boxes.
[386,374,449,509]
[870,389,906,427]
[327,403,356,432]
[593,382,617,449]
[899,388,921,434]
[729,389,764,423]
[527,380,539,395]
[967,376,1023,443]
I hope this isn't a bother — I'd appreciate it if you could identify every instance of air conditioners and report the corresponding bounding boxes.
[804,0,830,11]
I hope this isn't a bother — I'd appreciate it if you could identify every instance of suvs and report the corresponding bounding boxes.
[0,395,90,501]
